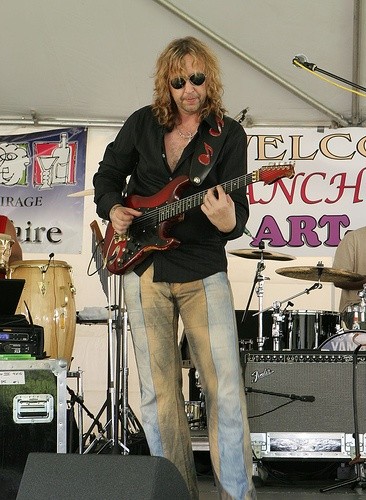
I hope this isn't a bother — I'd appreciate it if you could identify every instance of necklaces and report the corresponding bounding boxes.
[175,127,198,142]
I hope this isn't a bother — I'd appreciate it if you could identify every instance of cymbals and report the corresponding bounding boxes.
[275,266,365,283]
[227,247,297,262]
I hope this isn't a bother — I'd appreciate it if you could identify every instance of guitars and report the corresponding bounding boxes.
[101,159,299,275]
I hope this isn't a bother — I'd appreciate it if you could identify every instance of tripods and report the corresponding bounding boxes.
[76,309,147,456]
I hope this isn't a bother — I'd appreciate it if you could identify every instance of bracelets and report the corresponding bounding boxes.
[110,205,121,220]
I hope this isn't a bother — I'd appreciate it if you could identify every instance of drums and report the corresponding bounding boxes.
[318,329,366,352]
[0,233,12,269]
[182,400,207,430]
[5,258,77,373]
[279,310,342,352]
[194,337,255,394]
[344,304,366,330]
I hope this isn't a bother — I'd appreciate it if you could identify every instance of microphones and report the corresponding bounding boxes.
[288,302,294,306]
[49,253,54,257]
[292,54,308,67]
[296,396,315,402]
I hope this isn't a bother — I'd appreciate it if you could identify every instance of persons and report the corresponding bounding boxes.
[332,226,366,330]
[93,35,258,500]
[0,215,22,261]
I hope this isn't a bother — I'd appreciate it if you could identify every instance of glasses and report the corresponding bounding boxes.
[169,71,208,90]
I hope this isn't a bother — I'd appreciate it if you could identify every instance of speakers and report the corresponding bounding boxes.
[16,452,192,500]
[240,350,366,434]
